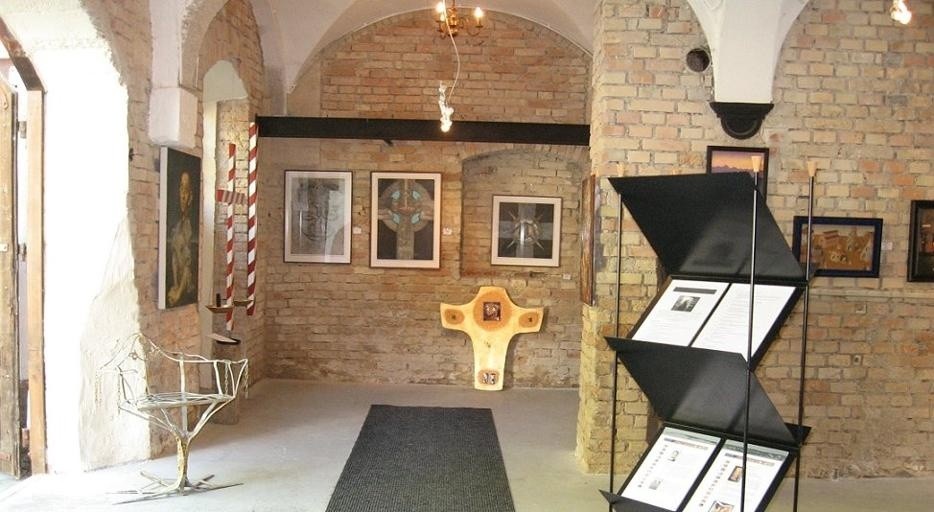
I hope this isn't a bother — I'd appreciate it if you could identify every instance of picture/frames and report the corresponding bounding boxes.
[283,168,442,271]
[906,199,934,283]
[489,194,563,268]
[791,214,882,279]
[705,145,770,202]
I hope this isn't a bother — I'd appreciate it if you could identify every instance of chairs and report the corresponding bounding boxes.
[97,331,249,506]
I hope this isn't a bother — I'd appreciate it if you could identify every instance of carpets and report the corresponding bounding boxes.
[325,404,516,511]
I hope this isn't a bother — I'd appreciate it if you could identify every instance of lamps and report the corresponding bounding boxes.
[889,0,913,27]
[432,1,485,133]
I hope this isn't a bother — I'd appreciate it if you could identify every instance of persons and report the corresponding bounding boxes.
[168,170,198,306]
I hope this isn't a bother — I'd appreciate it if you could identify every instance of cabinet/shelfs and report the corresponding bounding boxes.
[600,170,814,512]
